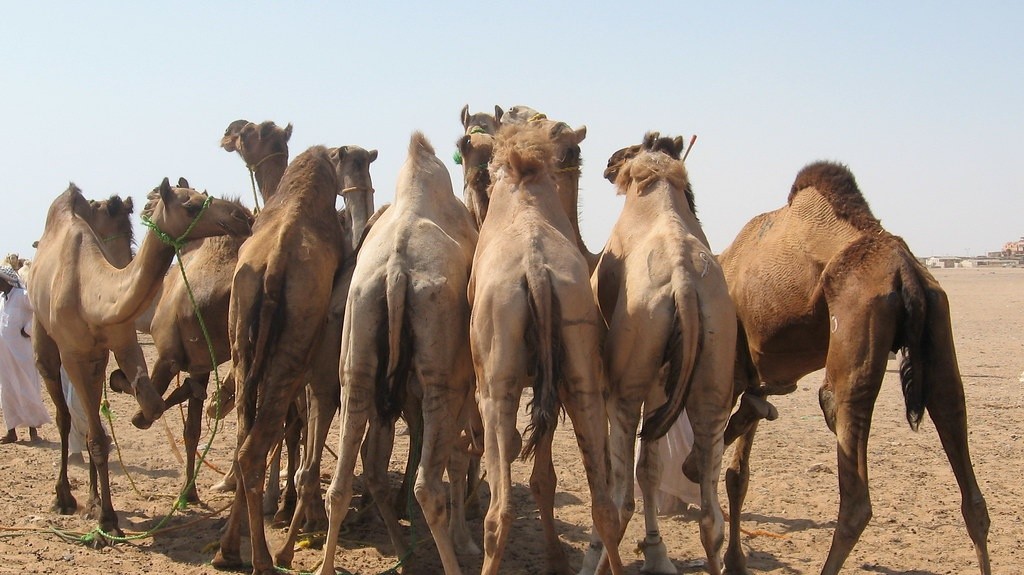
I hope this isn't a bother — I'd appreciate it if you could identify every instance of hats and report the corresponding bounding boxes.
[0,265,20,288]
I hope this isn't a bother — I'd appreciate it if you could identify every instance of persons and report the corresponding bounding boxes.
[634,406,701,515]
[0,251,52,445]
[60,360,113,464]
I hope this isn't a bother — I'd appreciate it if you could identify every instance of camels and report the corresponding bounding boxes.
[24,103,994,575]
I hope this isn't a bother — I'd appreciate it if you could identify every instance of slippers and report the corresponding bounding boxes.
[0,436,19,444]
[31,436,42,443]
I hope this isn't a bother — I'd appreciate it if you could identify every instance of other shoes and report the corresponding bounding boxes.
[53,453,86,466]
[106,435,112,445]
